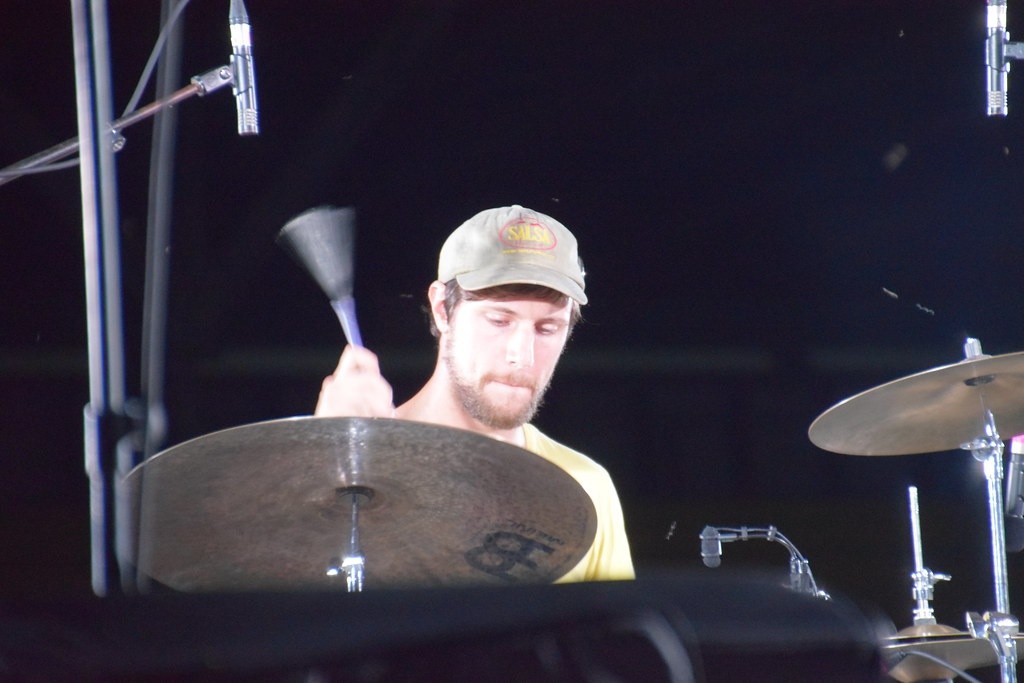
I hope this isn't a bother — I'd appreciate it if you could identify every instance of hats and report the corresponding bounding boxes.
[431,201,589,308]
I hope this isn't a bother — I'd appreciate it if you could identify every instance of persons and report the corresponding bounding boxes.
[317,202,639,585]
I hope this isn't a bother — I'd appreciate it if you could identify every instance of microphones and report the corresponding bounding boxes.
[985,0,1010,119]
[229,0,259,137]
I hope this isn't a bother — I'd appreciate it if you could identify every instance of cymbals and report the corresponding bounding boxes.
[879,620,1023,683]
[807,348,1022,458]
[118,414,597,586]
